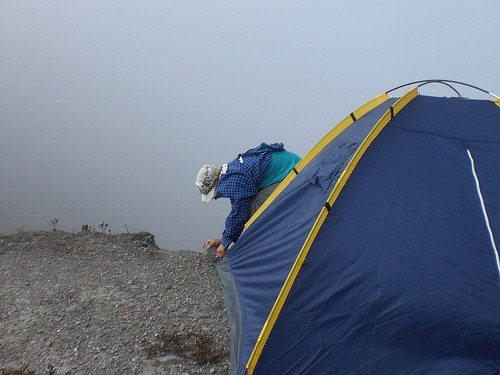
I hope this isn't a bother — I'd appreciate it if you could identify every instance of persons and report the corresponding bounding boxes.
[195,142,302,260]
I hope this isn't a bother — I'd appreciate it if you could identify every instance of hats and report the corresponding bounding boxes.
[196,164,228,203]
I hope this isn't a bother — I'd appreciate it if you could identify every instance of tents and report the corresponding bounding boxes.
[210,78,500,375]
